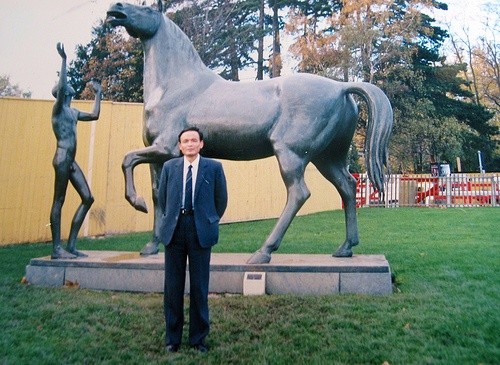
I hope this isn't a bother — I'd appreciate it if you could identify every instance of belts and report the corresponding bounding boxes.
[181,209,194,215]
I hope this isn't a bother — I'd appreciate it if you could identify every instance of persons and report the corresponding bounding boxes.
[49,41,106,260]
[155,125,229,358]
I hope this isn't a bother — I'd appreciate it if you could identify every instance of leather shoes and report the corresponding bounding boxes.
[166,344,180,352]
[195,345,207,352]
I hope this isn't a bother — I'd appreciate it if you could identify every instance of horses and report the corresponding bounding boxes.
[107,3,393,263]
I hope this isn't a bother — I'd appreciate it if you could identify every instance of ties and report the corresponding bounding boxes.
[184,164,193,215]
